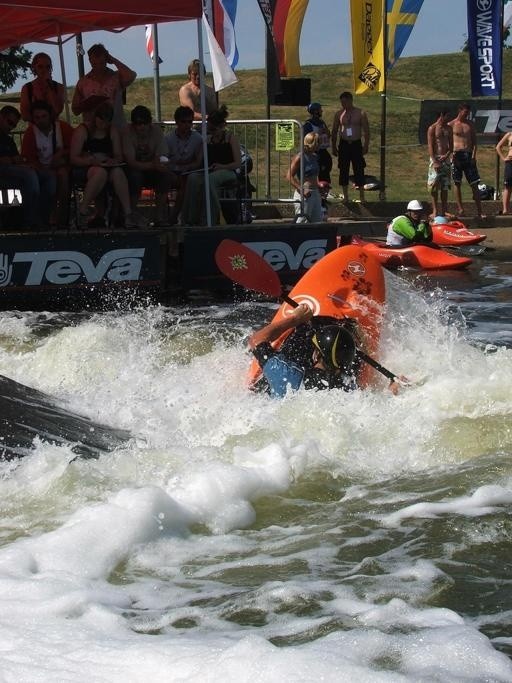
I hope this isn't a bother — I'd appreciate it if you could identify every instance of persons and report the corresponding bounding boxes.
[426,108,455,219]
[494,131,512,216]
[330,90,370,205]
[386,199,434,247]
[20,53,66,123]
[177,58,218,120]
[71,44,136,125]
[248,300,406,398]
[285,129,323,223]
[0,100,243,229]
[446,103,487,219]
[302,102,333,185]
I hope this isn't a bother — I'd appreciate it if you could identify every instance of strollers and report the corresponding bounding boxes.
[237,145,257,223]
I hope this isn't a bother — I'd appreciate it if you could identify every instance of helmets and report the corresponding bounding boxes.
[312,325,357,369]
[433,216,447,224]
[308,103,320,114]
[407,200,423,211]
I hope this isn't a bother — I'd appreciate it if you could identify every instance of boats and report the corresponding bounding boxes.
[428,220,488,248]
[344,232,470,274]
[244,243,386,396]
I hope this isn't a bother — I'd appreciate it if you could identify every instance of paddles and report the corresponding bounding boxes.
[215,239,412,395]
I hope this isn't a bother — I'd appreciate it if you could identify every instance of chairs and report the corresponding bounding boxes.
[73,181,242,228]
